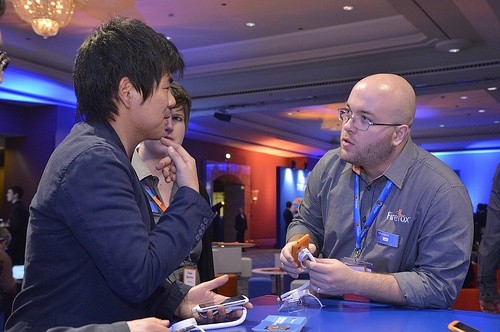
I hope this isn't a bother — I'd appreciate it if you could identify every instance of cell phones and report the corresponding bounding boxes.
[196,295,249,312]
[291,234,309,268]
[448,320,482,332]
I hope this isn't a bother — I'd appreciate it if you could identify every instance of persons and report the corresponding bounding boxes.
[0,185,31,267]
[234,207,248,253]
[283,201,294,232]
[474,203,488,242]
[0,228,22,316]
[7,14,252,331]
[278,74,473,310]
[478,161,500,314]
[46,316,171,332]
[130,81,218,329]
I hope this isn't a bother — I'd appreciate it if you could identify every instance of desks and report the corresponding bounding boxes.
[252,268,288,296]
[191,295,500,332]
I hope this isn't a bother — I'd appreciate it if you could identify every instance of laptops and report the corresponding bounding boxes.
[12,265,26,281]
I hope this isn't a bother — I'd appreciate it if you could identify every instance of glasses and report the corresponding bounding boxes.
[338,107,402,131]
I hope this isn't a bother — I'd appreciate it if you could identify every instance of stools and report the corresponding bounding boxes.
[283,273,310,294]
[248,276,273,298]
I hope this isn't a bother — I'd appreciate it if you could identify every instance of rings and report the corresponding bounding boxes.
[317,287,320,294]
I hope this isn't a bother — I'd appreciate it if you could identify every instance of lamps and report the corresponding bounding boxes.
[12,0,76,39]
[214,112,232,122]
